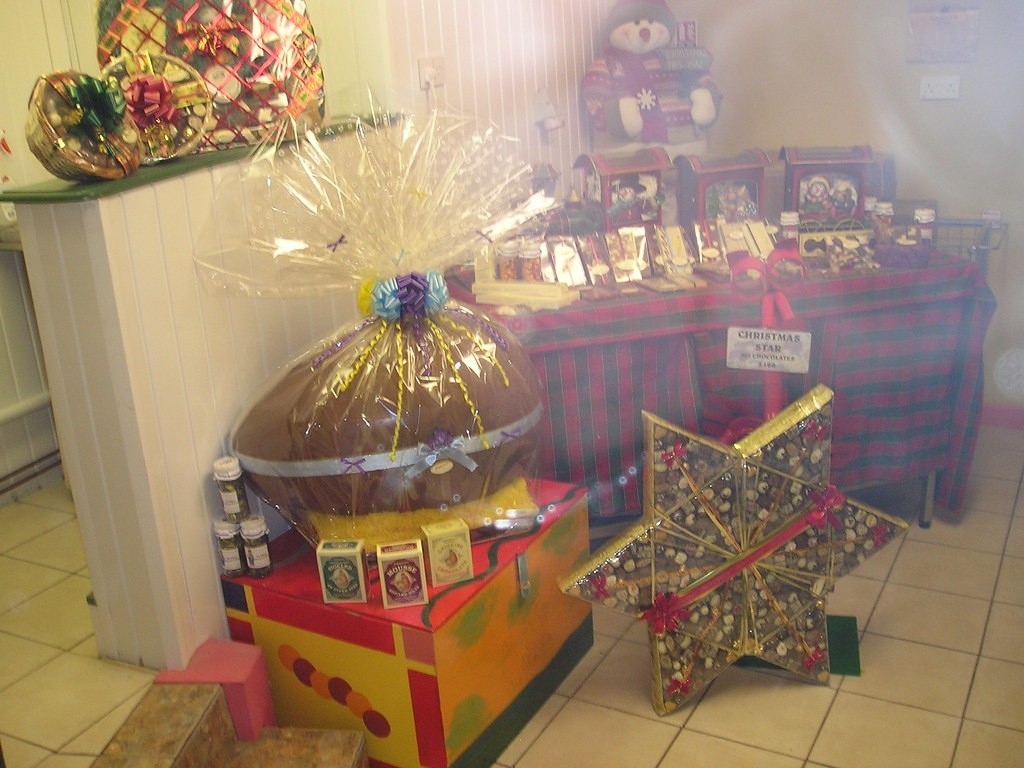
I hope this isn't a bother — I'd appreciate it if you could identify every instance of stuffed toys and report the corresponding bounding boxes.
[583,0,722,166]
[591,173,859,227]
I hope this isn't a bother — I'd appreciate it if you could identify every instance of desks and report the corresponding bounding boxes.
[469,237,998,531]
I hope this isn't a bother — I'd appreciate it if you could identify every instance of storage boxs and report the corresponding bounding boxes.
[220,481,592,768]
[570,144,871,226]
